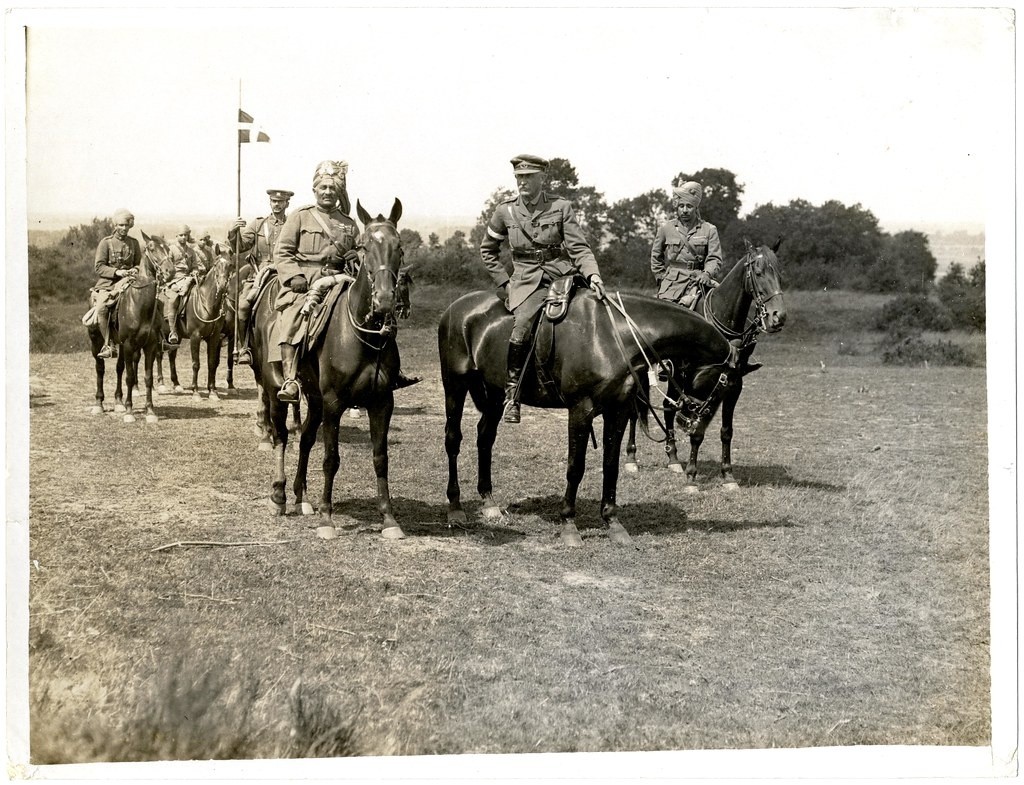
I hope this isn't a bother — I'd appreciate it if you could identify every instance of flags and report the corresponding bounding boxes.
[238,110,271,143]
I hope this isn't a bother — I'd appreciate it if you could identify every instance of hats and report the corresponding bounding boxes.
[266,189,294,201]
[509,155,548,175]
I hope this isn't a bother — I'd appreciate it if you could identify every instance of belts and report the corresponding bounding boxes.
[668,259,707,271]
[300,260,345,271]
[511,248,562,265]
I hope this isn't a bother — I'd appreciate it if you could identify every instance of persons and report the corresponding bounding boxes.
[480,154,604,422]
[164,224,218,344]
[272,159,416,399]
[228,189,294,364]
[94,208,141,357]
[396,269,414,319]
[649,181,723,381]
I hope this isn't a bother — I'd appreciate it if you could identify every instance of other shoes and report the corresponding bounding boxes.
[743,362,762,376]
[658,360,671,381]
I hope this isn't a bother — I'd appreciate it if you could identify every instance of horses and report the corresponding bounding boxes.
[623,235,790,495]
[87,228,177,422]
[154,242,255,401]
[436,289,765,549]
[249,197,410,542]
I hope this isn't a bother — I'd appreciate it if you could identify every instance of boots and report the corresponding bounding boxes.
[168,308,178,343]
[236,317,252,365]
[504,341,525,424]
[278,342,300,401]
[392,372,422,387]
[97,314,118,358]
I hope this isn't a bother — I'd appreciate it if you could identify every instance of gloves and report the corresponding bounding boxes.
[290,275,308,293]
[342,249,361,266]
[696,272,711,284]
